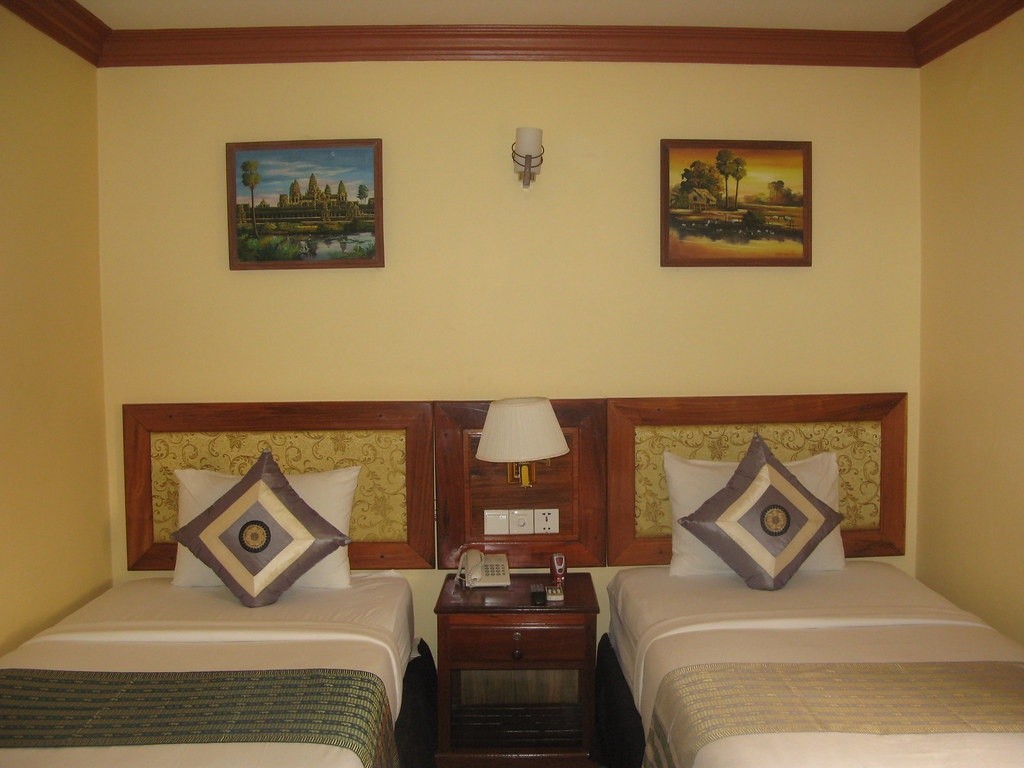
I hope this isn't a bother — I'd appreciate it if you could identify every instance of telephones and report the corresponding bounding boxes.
[463,547,511,588]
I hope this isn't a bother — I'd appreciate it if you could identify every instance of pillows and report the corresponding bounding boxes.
[676,432,845,591]
[663,451,845,576]
[168,451,353,608]
[170,466,360,590]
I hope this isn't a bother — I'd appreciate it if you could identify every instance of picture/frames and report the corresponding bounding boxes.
[226,138,385,271]
[659,138,813,267]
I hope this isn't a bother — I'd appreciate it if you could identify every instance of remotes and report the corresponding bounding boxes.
[531,583,564,605]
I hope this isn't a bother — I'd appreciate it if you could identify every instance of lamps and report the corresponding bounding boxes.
[512,128,544,187]
[476,396,570,491]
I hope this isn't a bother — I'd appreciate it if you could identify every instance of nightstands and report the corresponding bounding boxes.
[433,573,600,768]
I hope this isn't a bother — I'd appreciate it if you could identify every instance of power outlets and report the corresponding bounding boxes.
[535,508,559,534]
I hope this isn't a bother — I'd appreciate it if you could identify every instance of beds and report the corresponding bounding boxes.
[0,573,414,768]
[606,559,1024,768]
